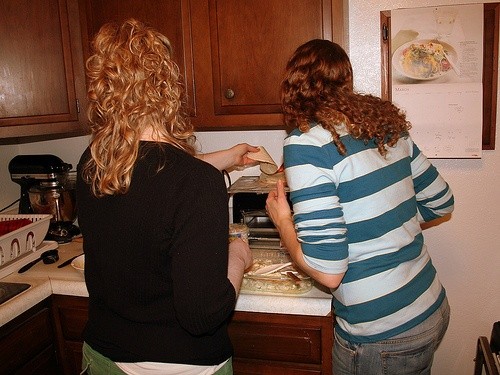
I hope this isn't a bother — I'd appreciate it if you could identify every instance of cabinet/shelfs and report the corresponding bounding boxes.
[0,0,349,139]
[0,294,335,375]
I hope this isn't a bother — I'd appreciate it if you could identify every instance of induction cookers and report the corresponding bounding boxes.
[0,278,30,307]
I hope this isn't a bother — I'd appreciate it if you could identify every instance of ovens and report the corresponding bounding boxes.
[228,175,296,245]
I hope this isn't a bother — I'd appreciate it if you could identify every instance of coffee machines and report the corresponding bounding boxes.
[8,154,76,243]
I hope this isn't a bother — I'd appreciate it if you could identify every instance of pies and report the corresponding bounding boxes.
[246,146,278,175]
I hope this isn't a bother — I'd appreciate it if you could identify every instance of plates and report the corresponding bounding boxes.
[391,39,458,79]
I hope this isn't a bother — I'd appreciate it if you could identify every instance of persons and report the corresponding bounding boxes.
[75,18,261,375]
[264,38,455,375]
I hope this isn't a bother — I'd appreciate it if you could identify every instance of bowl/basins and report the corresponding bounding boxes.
[70,254,85,274]
[228,227,250,245]
[27,181,77,228]
